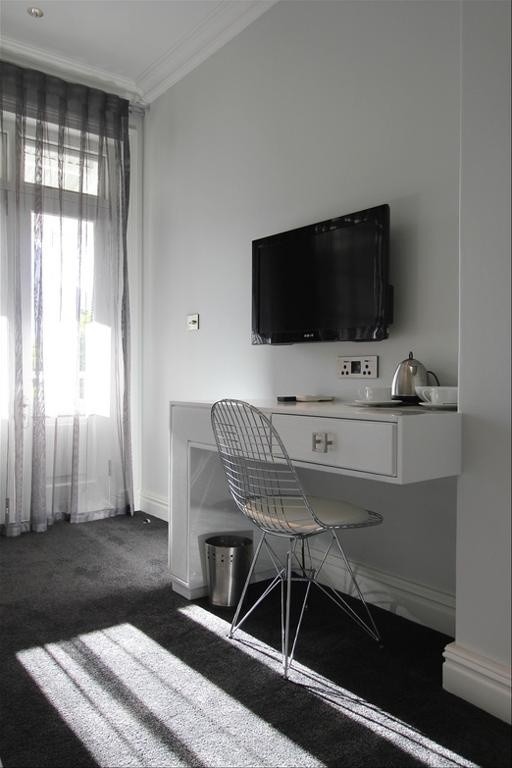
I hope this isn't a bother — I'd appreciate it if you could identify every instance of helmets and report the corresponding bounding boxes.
[353,400,403,407]
[418,401,457,410]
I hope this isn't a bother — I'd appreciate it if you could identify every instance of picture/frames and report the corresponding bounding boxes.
[167,398,461,604]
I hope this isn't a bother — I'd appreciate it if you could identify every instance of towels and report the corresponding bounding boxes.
[205,536,252,607]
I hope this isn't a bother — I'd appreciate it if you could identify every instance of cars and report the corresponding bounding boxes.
[359,386,392,402]
[414,386,436,403]
[422,386,458,404]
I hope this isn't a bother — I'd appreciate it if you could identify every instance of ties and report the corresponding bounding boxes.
[250,204,394,346]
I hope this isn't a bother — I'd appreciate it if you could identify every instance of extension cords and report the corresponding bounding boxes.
[390,351,439,406]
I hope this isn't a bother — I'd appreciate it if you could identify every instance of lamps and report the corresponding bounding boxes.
[210,399,383,683]
